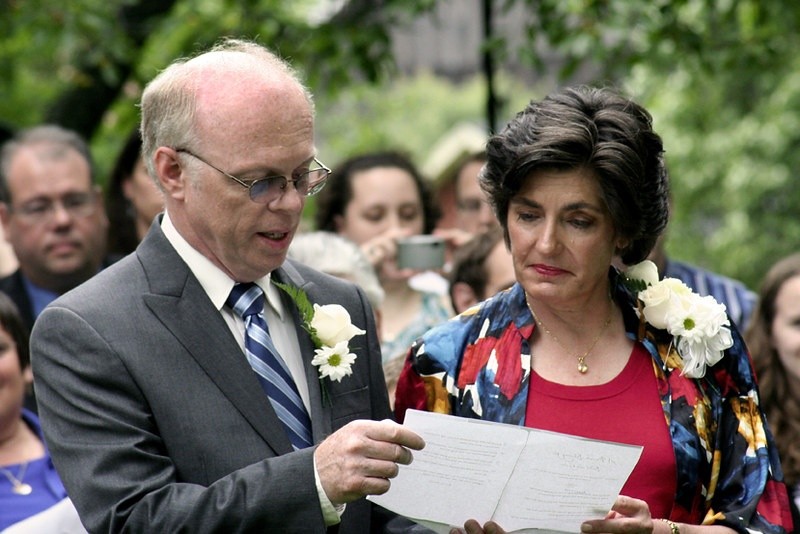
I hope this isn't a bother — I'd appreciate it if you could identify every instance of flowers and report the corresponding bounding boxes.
[622,258,735,380]
[269,279,367,410]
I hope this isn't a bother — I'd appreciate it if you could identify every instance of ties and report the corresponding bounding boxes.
[228,279,315,453]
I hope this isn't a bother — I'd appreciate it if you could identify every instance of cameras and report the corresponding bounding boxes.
[396,237,447,270]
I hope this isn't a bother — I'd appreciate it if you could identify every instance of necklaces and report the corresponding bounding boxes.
[0,444,32,496]
[524,292,612,375]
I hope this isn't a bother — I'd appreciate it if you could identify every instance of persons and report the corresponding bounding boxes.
[1,38,799,534]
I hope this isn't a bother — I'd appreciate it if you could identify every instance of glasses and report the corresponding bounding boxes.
[174,147,330,201]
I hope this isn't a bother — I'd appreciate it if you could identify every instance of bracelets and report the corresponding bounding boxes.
[661,518,680,534]
